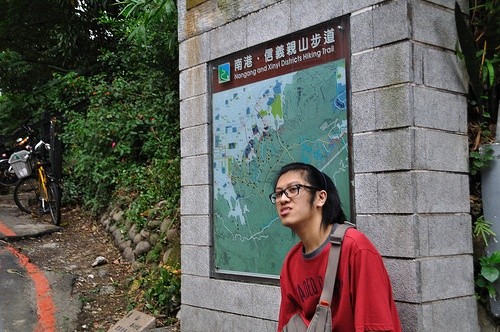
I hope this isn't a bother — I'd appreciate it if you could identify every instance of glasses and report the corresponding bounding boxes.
[269,184,321,204]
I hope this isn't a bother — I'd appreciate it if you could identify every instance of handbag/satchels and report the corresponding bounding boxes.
[283,304,332,332]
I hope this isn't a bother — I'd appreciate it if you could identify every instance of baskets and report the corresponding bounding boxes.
[8,150,32,178]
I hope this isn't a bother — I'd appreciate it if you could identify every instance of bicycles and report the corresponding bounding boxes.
[0,157,18,186]
[2,131,61,226]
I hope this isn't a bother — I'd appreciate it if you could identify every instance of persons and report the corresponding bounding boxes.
[269,162,402,332]
